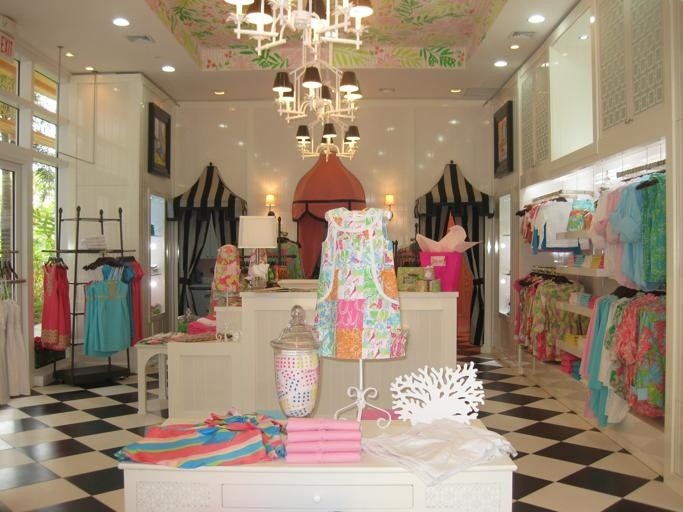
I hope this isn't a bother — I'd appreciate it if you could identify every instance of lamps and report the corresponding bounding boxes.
[385,194,394,221]
[222,0,373,164]
[238,216,278,290]
[264,193,274,217]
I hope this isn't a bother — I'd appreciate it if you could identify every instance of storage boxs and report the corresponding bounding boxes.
[419,251,464,292]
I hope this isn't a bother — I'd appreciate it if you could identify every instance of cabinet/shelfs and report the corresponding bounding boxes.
[555,231,609,359]
[118,280,517,512]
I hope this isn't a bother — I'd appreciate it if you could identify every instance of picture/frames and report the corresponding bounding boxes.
[493,100,513,179]
[148,102,171,178]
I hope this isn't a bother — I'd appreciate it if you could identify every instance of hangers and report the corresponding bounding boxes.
[516,191,567,217]
[82,249,136,271]
[277,232,301,248]
[610,287,666,298]
[45,250,68,271]
[520,265,573,288]
[0,250,18,279]
[621,160,666,189]
